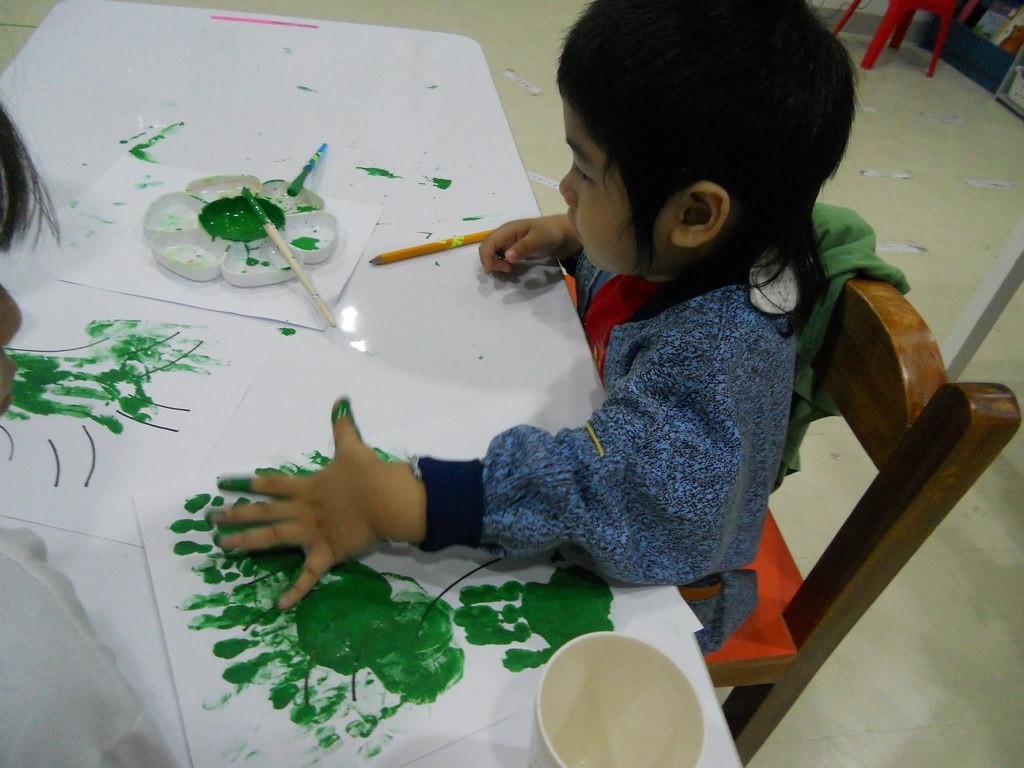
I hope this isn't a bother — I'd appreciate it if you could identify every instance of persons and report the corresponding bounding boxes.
[209,1,858,657]
[1,104,57,417]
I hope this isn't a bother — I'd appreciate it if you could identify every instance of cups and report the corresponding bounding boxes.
[527,632,704,768]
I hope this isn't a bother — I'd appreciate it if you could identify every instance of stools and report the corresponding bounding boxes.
[831,0,957,78]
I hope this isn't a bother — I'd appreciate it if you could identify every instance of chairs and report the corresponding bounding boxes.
[690,208,1022,767]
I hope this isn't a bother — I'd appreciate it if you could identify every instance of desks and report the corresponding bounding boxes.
[0,0,741,768]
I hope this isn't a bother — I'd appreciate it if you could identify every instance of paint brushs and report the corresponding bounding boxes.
[237,184,340,330]
[289,142,330,195]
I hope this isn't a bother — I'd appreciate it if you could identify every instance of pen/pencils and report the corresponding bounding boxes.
[369,229,494,269]
